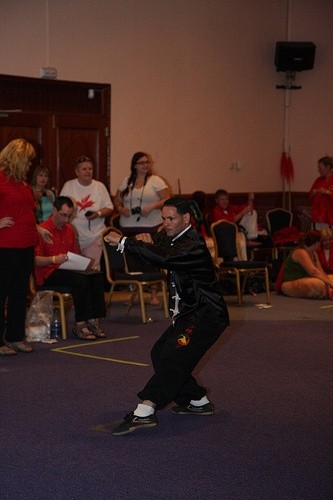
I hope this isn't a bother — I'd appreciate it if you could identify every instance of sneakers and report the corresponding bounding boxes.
[110,410,158,436]
[172,402,216,416]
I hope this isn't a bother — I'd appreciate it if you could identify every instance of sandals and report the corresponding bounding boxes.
[88,320,108,338]
[72,324,97,340]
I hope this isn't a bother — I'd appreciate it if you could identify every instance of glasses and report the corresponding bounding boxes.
[135,160,150,164]
[76,157,91,162]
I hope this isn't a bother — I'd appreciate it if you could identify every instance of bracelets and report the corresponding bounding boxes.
[49,256,55,263]
[97,211,101,218]
[116,235,127,253]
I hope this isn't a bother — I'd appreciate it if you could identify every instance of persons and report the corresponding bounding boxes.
[60,157,114,272]
[103,195,230,435]
[274,157,333,299]
[114,152,171,306]
[191,189,254,240]
[33,196,106,340]
[0,139,53,355]
[30,165,55,224]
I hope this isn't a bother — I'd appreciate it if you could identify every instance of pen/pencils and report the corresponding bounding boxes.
[64,256,68,259]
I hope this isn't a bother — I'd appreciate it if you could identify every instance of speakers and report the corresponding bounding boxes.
[273,41,317,71]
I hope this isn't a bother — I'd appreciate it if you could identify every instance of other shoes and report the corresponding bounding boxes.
[6,341,34,353]
[149,297,161,306]
[0,346,18,355]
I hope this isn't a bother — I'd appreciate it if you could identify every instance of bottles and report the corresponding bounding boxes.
[50,307,61,340]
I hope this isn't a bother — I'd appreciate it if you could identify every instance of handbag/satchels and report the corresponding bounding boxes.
[238,210,258,239]
[23,291,58,344]
[271,227,302,246]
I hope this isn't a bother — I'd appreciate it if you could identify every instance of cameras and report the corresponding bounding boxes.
[131,207,141,214]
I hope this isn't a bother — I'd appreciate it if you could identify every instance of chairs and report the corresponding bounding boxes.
[211,205,303,307]
[29,275,73,341]
[100,213,172,324]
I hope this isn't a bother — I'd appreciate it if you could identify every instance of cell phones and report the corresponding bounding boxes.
[85,211,91,216]
